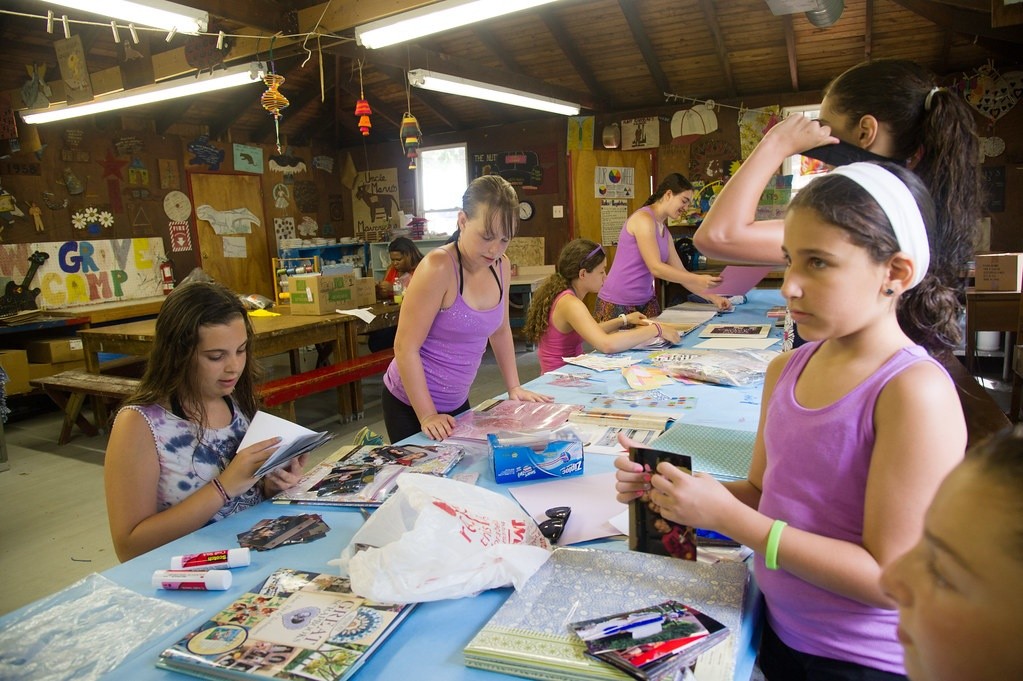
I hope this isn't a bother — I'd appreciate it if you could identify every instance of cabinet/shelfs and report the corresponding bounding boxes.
[279,242,369,277]
[370,238,450,283]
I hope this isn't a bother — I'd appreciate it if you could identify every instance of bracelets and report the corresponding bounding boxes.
[212,478,230,502]
[619,314,627,326]
[765,520,789,570]
[653,322,662,337]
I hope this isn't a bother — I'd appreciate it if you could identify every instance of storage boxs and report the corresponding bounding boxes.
[289,273,357,316]
[973,253,1018,292]
[27,361,88,391]
[0,350,31,395]
[488,431,583,484]
[22,337,86,364]
[353,277,377,307]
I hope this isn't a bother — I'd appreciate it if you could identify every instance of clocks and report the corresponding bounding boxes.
[518,200,536,221]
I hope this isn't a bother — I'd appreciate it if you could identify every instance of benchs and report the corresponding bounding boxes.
[29,372,144,444]
[252,348,395,412]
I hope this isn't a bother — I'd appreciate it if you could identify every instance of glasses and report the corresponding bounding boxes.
[538,507,571,545]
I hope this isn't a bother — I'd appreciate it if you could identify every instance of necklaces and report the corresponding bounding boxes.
[657,220,662,227]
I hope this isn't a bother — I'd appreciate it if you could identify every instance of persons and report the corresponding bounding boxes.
[104,282,310,564]
[616,161,969,681]
[382,175,555,444]
[593,173,732,333]
[692,57,979,350]
[723,328,749,334]
[881,434,1023,681]
[639,489,696,560]
[317,449,427,497]
[525,240,681,372]
[368,237,425,353]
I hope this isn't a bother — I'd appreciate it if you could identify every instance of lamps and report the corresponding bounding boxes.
[41,0,209,37]
[354,0,560,50]
[406,51,581,116]
[18,61,268,126]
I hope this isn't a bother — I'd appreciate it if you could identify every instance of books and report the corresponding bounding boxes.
[621,301,736,350]
[272,442,465,507]
[462,398,757,480]
[768,306,787,317]
[464,546,751,681]
[155,568,417,681]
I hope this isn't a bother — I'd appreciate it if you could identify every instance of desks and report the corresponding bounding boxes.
[0,295,790,681]
[966,292,1023,377]
[509,275,555,353]
